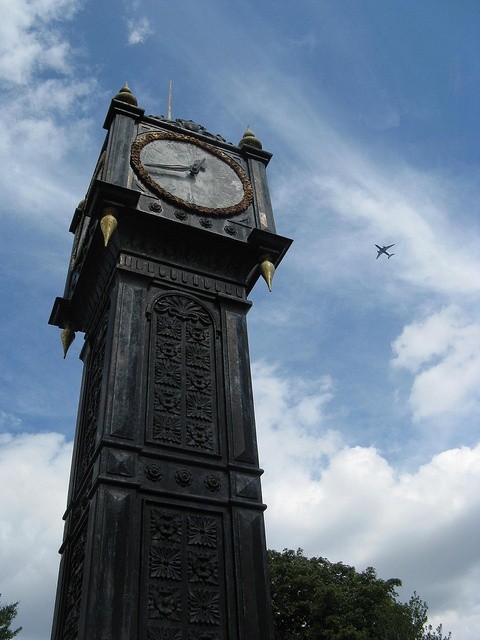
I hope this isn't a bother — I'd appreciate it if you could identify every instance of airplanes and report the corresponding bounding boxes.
[375,243,395,260]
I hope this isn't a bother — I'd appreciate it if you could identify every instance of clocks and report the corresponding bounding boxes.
[130,131,253,215]
